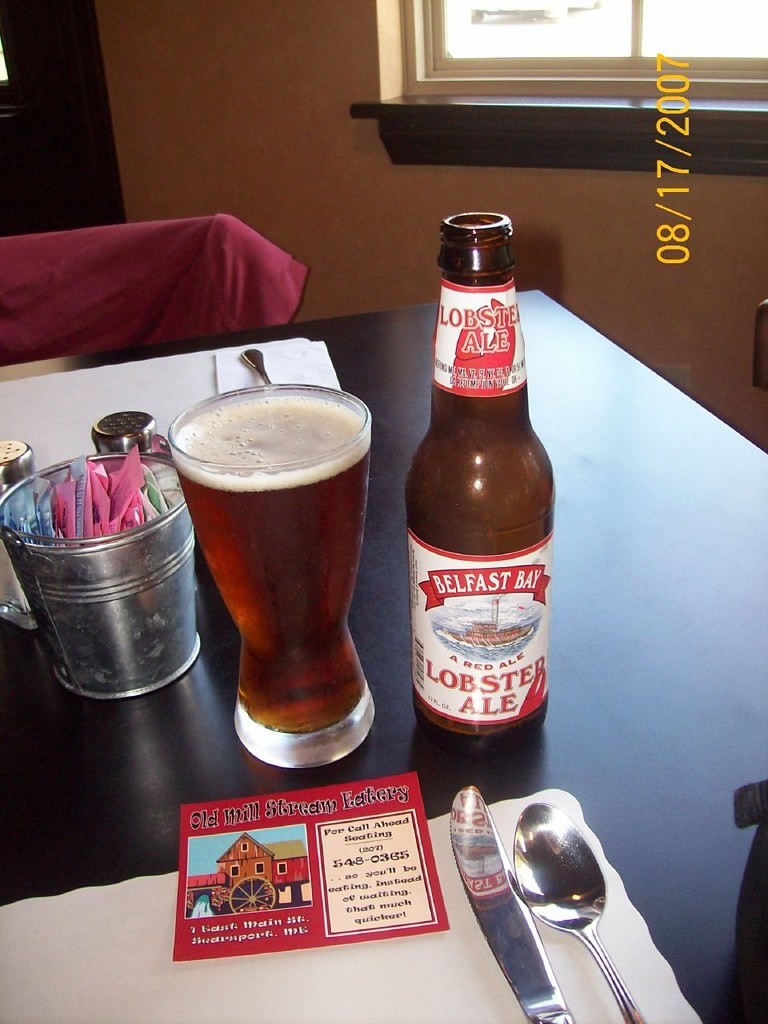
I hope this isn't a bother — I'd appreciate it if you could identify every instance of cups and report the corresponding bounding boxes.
[167,385,374,768]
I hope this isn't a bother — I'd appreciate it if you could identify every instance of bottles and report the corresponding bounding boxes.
[406,212,555,757]
[0,441,38,629]
[92,412,157,454]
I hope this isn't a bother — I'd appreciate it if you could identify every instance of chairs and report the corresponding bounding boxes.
[0,213,313,365]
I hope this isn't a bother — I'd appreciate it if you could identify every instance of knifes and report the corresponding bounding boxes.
[450,786,576,1024]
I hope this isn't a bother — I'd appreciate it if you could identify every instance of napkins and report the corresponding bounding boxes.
[0,790,701,1024]
[216,339,342,396]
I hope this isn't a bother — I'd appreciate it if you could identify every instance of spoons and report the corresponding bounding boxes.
[514,803,646,1024]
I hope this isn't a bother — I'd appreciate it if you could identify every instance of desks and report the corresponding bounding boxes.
[0,290,768,1024]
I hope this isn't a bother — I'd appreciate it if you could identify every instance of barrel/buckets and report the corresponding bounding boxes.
[0,434,200,698]
